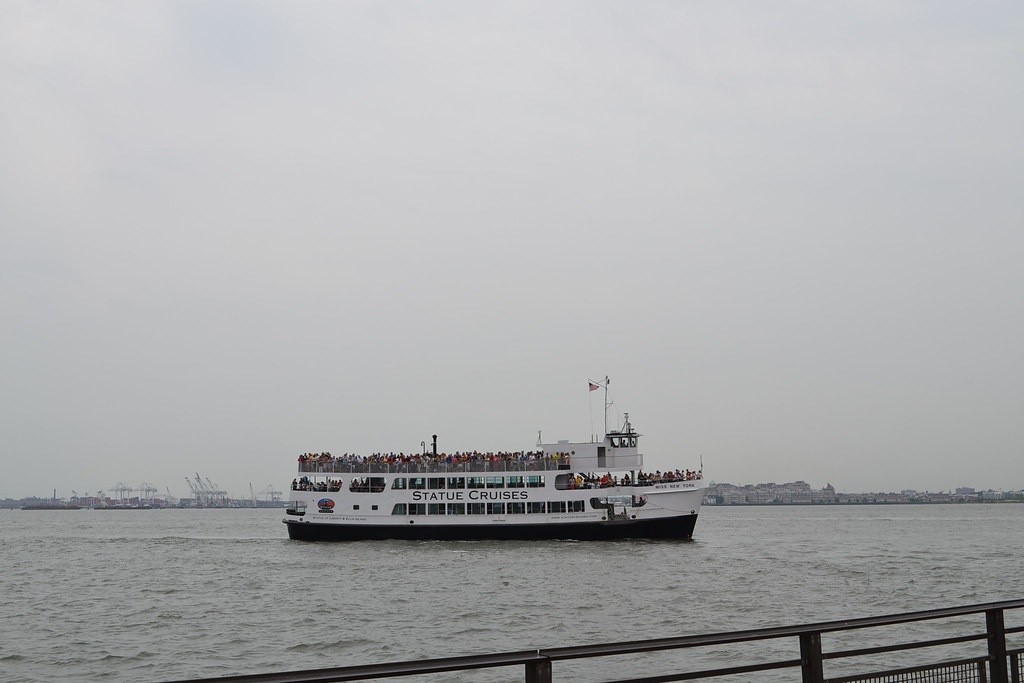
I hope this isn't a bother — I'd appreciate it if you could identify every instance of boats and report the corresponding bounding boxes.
[281,375,704,541]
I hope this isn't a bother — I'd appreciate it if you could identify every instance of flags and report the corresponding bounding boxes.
[589,383,599,390]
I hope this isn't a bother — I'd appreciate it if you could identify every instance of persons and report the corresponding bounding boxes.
[567,469,702,490]
[293,477,363,491]
[298,449,571,474]
[622,440,627,446]
